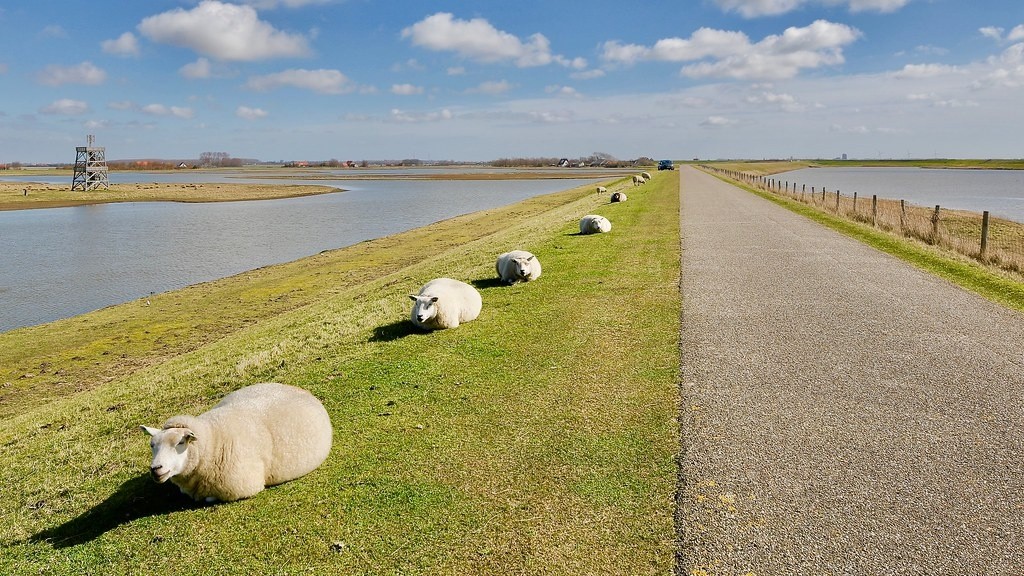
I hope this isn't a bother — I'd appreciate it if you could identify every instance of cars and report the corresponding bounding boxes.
[657,159,674,170]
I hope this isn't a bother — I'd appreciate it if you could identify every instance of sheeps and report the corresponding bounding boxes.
[642,172,651,179]
[410,278,480,329]
[597,187,606,193]
[611,192,627,203]
[579,215,611,234]
[495,251,542,281]
[633,176,645,185]
[141,381,334,506]
